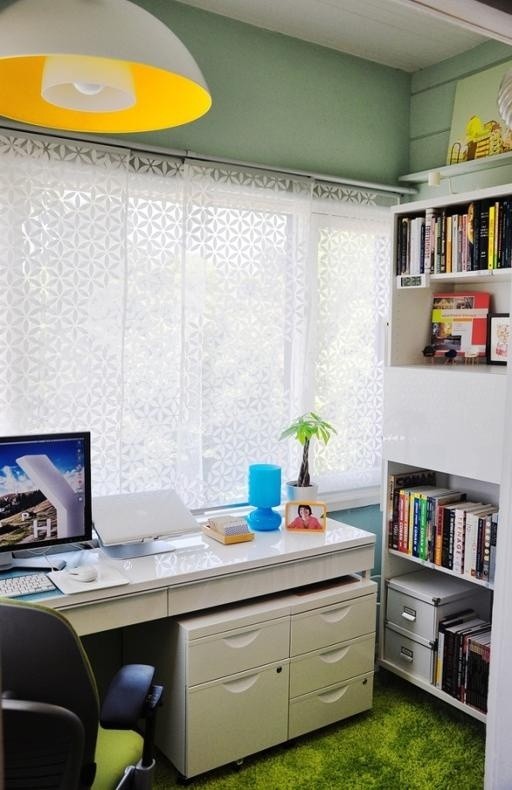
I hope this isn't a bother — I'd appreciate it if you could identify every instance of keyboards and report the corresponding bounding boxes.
[0,573,57,600]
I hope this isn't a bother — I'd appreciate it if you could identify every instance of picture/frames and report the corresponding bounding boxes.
[285,500,327,533]
[486,312,509,366]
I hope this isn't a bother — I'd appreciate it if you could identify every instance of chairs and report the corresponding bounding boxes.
[0,597,164,790]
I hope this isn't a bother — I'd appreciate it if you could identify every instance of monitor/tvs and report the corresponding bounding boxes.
[0,430,92,571]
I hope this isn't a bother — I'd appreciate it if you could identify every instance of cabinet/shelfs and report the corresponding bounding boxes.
[379,182,512,724]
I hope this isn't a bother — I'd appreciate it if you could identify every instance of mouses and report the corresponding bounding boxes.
[67,562,99,583]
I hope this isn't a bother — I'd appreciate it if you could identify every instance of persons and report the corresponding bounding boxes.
[288,505,322,529]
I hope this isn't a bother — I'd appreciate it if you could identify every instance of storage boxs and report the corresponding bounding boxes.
[383,568,478,685]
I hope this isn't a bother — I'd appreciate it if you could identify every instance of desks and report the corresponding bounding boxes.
[0,508,376,638]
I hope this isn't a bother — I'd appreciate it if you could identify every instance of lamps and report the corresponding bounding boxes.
[427,171,453,194]
[0,1,213,134]
[246,464,282,531]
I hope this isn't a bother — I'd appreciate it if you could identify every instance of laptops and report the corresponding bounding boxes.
[90,488,202,548]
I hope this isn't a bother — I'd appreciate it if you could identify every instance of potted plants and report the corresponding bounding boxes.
[277,412,338,502]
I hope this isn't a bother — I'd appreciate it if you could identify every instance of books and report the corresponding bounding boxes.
[395,199,511,275]
[432,608,490,715]
[388,470,498,583]
